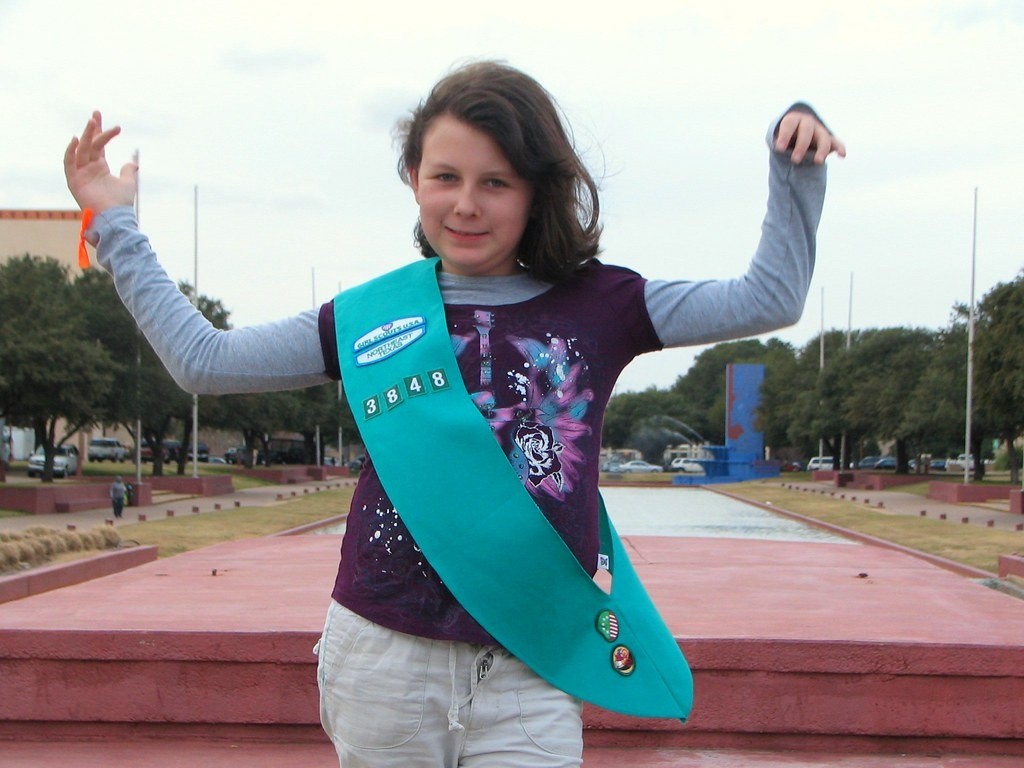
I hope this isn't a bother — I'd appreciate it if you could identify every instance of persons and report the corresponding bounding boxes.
[331,457,335,465]
[126,481,135,505]
[65,62,846,768]
[109,475,126,518]
[914,455,930,474]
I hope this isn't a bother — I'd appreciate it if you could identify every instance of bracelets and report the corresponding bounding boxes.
[78,208,92,269]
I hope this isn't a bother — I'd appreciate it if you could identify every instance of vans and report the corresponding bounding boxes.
[806,456,842,471]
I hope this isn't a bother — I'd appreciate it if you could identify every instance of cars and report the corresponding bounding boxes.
[87,438,126,464]
[602,460,664,473]
[132,440,227,464]
[26,442,80,479]
[672,457,704,472]
[848,456,976,472]
[349,454,365,468]
[323,457,340,466]
